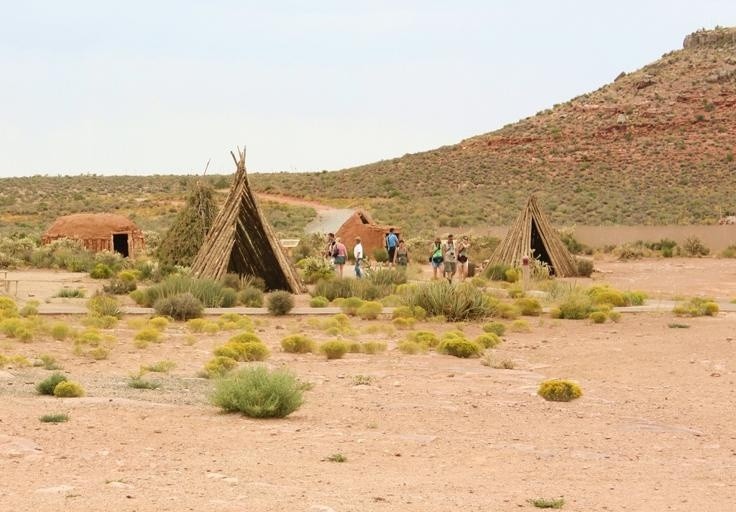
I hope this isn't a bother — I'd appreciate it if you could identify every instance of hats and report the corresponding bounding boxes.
[355,236,361,241]
[434,237,441,243]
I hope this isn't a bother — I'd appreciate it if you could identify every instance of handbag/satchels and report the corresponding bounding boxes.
[332,248,339,258]
[429,256,433,262]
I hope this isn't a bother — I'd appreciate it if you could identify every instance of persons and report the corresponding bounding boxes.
[335,237,348,280]
[443,235,458,284]
[386,227,399,263]
[327,233,338,265]
[393,240,412,270]
[429,237,445,279]
[353,236,363,277]
[456,236,470,281]
